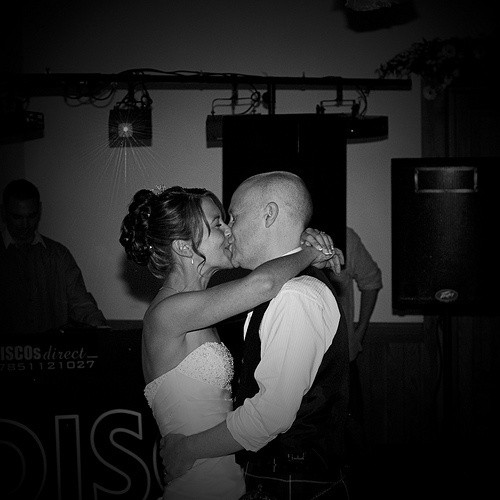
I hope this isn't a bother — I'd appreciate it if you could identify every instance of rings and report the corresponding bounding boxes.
[318,231,325,234]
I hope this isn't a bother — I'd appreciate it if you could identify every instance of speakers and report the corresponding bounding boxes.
[391,192,500,318]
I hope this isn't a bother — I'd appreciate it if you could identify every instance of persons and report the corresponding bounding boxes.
[119,171,353,500]
[0,178,105,348]
[339,225,383,437]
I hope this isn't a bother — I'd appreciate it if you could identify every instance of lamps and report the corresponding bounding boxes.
[0,100,43,145]
[317,89,388,144]
[109,85,151,148]
[206,89,256,147]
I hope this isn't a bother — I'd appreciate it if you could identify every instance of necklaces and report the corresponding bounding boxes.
[160,286,180,294]
